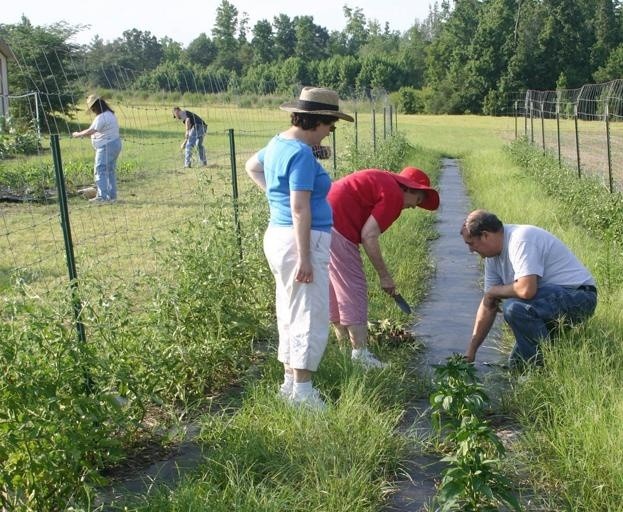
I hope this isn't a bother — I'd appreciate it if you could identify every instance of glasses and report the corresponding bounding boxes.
[330,125,335,132]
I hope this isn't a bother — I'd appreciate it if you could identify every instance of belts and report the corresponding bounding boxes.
[578,285,596,292]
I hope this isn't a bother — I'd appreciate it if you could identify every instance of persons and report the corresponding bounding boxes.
[245,86,354,413]
[459,209,598,380]
[72,94,122,205]
[326,166,440,370]
[172,106,208,168]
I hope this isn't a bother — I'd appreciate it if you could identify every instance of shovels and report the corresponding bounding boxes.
[393,293,411,316]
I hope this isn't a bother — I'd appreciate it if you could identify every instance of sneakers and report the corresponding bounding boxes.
[88,197,103,204]
[351,352,381,368]
[288,388,326,410]
[279,384,292,397]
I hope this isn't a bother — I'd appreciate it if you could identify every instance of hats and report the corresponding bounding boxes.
[86,95,102,112]
[172,107,178,118]
[278,86,354,122]
[385,167,439,210]
